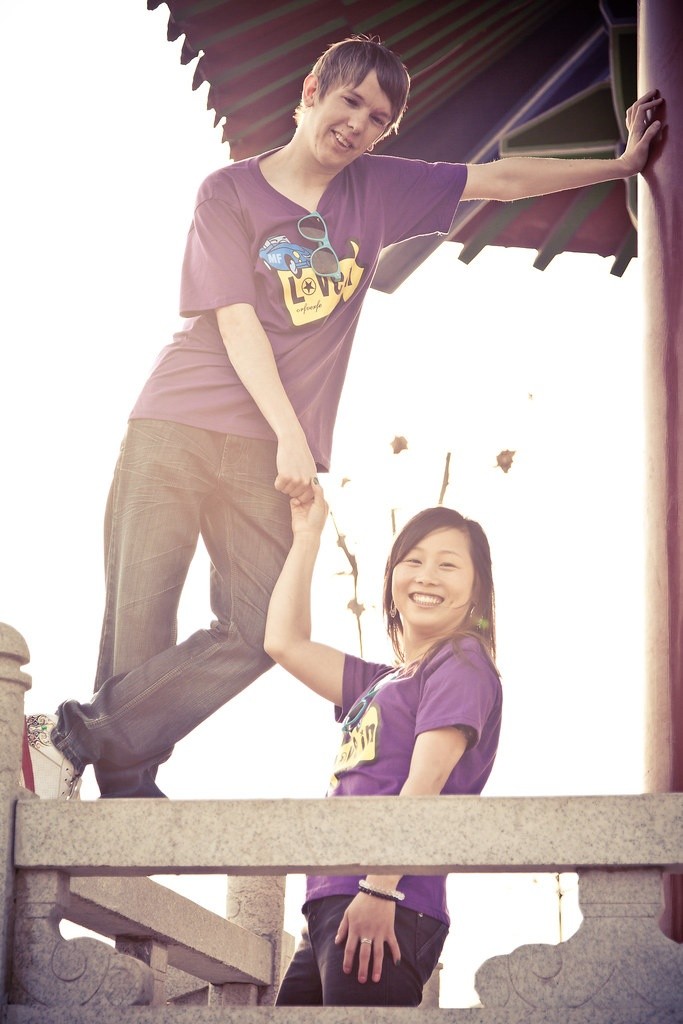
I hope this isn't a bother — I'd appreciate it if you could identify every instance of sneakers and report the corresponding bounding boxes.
[23,715,81,801]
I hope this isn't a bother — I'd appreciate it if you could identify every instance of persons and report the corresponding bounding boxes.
[20,33,666,800]
[261,479,505,1005]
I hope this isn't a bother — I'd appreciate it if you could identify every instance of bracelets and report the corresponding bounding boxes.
[357,879,406,901]
[357,886,399,902]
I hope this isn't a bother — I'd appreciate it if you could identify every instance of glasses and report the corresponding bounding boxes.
[342,671,401,730]
[299,210,343,282]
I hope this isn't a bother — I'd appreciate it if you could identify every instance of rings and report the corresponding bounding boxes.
[361,939,372,945]
